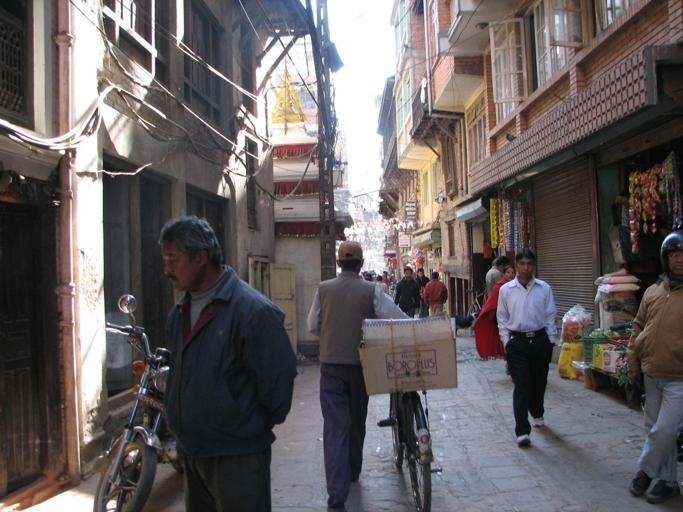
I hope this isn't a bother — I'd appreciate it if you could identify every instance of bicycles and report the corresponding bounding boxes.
[375,390,442,512]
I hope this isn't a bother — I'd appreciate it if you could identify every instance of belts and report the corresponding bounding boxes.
[509,327,546,338]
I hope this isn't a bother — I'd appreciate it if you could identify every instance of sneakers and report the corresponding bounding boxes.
[325,503,345,512]
[515,432,531,448]
[628,468,652,497]
[644,478,680,504]
[349,470,359,482]
[530,416,546,428]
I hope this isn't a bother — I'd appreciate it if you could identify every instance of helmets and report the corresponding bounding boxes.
[658,229,683,273]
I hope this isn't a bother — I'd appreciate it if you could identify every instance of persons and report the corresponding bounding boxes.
[625,229,683,503]
[486,257,510,299]
[474,266,516,376]
[496,246,558,447]
[156,216,299,512]
[362,271,448,319]
[307,240,413,512]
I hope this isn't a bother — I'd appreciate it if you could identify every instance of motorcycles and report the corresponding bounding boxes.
[92,294,187,511]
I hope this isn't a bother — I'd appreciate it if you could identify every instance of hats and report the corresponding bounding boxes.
[336,239,364,262]
